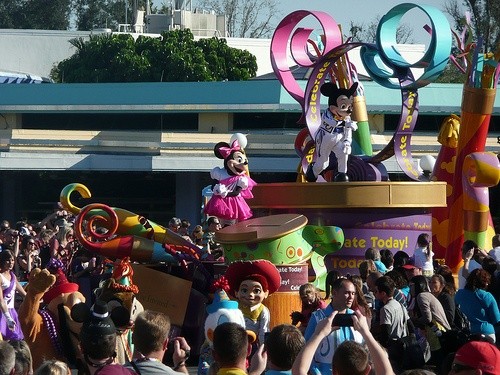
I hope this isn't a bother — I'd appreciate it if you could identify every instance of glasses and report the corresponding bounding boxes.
[451,362,476,374]
[212,223,219,225]
[409,282,415,286]
[28,242,35,245]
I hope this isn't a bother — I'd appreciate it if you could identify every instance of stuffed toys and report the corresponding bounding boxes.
[204,141,257,225]
[18,265,89,375]
[90,260,144,367]
[306,81,358,182]
[204,282,256,360]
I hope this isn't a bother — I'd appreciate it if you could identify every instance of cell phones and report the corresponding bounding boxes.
[332,314,357,326]
[474,249,478,254]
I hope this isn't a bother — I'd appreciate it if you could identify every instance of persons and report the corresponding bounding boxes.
[229,257,282,344]
[168,215,220,242]
[401,268,500,375]
[72,299,138,375]
[0,210,79,375]
[367,270,415,375]
[305,271,366,375]
[292,308,394,375]
[208,321,250,375]
[125,311,190,375]
[292,284,327,335]
[250,325,309,375]
[359,231,500,305]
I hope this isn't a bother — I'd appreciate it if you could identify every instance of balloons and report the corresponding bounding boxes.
[229,133,247,150]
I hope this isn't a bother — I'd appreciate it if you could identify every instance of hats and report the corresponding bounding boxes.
[55,218,74,229]
[455,341,500,375]
[402,257,423,270]
[82,310,116,335]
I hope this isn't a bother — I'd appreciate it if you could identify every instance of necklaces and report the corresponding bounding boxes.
[38,308,63,362]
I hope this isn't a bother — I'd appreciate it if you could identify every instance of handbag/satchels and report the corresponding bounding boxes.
[402,337,432,365]
[440,330,467,351]
